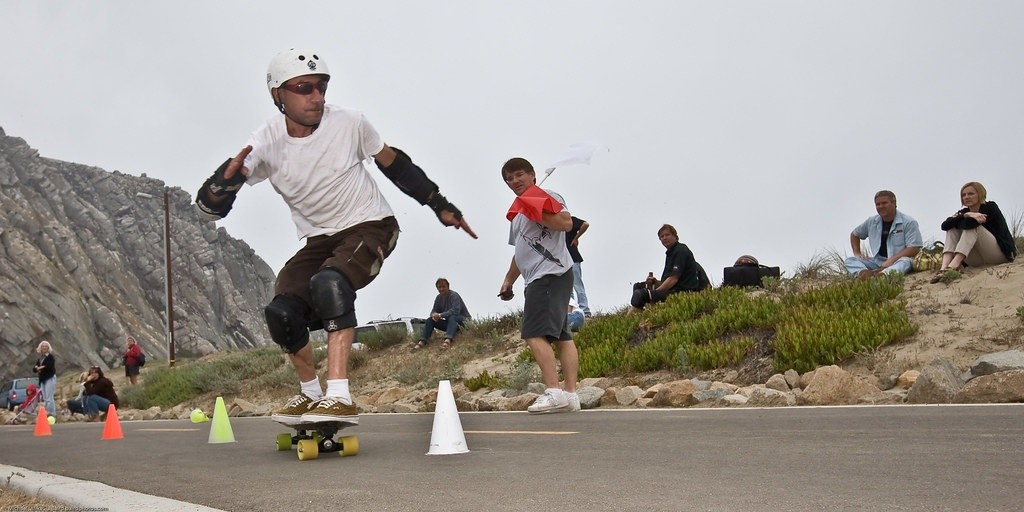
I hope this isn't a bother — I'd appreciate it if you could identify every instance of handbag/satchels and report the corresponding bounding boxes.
[913,241,944,271]
[723,265,782,289]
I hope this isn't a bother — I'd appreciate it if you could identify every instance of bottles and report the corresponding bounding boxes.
[645,272,655,290]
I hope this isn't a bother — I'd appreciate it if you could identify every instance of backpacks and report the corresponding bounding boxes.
[136,351,146,366]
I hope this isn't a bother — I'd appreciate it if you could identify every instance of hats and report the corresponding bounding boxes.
[734,254,759,268]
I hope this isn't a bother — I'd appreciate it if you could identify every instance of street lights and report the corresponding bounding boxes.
[136,186,185,369]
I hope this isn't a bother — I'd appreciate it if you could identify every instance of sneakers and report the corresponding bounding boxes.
[527,389,580,412]
[271,393,317,421]
[299,396,359,424]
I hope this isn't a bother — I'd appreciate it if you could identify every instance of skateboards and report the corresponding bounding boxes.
[271,412,360,461]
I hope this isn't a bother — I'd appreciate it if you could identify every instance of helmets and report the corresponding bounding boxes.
[266,45,331,94]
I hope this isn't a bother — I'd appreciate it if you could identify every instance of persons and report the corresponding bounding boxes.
[626,224,707,320]
[33,341,57,424]
[413,278,472,351]
[59,366,119,422]
[192,41,477,422]
[10,385,37,421]
[843,190,923,284]
[121,337,142,386]
[930,182,1017,285]
[500,157,583,414]
[566,217,592,320]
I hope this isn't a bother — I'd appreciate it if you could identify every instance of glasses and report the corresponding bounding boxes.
[90,366,99,369]
[282,81,328,95]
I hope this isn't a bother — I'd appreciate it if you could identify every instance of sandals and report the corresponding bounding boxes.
[440,340,451,349]
[410,343,425,350]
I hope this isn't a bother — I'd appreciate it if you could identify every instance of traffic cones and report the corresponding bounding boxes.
[33,407,52,436]
[208,396,238,444]
[189,409,209,423]
[101,403,124,439]
[424,379,471,456]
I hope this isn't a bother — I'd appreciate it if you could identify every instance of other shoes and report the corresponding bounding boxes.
[930,266,958,284]
[86,414,101,422]
[857,270,888,282]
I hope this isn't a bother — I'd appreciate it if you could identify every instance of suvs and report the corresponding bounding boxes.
[0,377,40,411]
[353,318,438,351]
